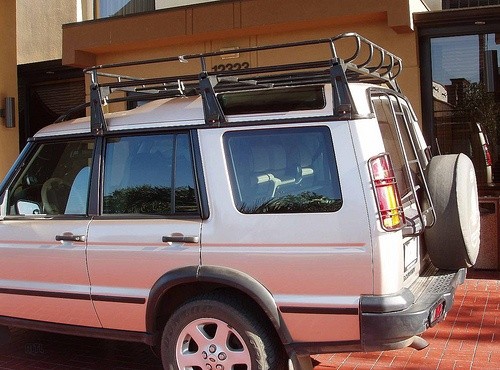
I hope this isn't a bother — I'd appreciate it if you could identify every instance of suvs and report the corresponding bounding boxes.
[433,107,495,189]
[0,31,481,370]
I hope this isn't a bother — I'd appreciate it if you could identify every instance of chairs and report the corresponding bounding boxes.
[247,166,327,207]
